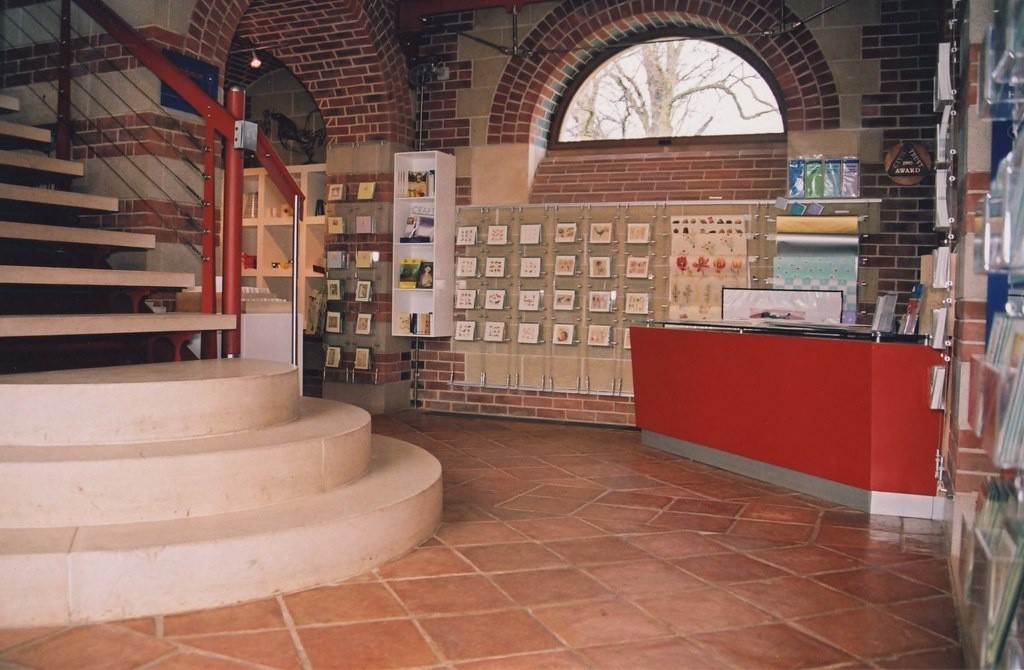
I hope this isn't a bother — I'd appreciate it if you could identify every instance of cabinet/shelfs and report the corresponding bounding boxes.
[218,163,325,329]
[939,0,1024,670]
[392,151,457,339]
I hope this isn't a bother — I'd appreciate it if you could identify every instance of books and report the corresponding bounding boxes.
[395,311,433,335]
[399,258,433,289]
[871,0,1024,670]
[407,170,429,197]
[403,205,435,243]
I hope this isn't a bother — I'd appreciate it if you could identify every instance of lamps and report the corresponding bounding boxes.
[250,52,261,66]
